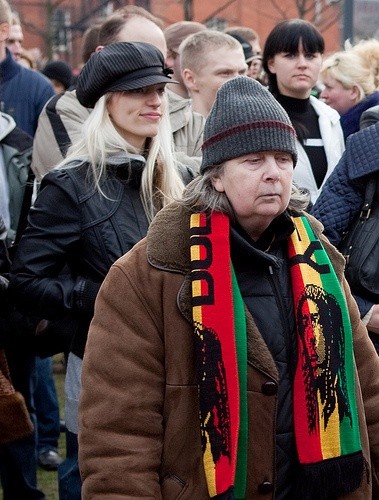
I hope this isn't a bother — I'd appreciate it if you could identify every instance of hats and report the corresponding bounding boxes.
[75,41,180,110]
[231,34,263,64]
[200,76,297,175]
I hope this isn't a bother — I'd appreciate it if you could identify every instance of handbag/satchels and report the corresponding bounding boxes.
[337,207,379,305]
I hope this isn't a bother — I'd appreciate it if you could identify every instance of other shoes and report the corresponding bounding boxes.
[38,450,65,472]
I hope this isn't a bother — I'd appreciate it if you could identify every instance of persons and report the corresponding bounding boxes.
[7,42,178,500]
[262,19,346,204]
[0,1,72,500]
[309,121,378,353]
[161,22,262,174]
[30,5,167,180]
[319,36,379,138]
[77,78,379,500]
